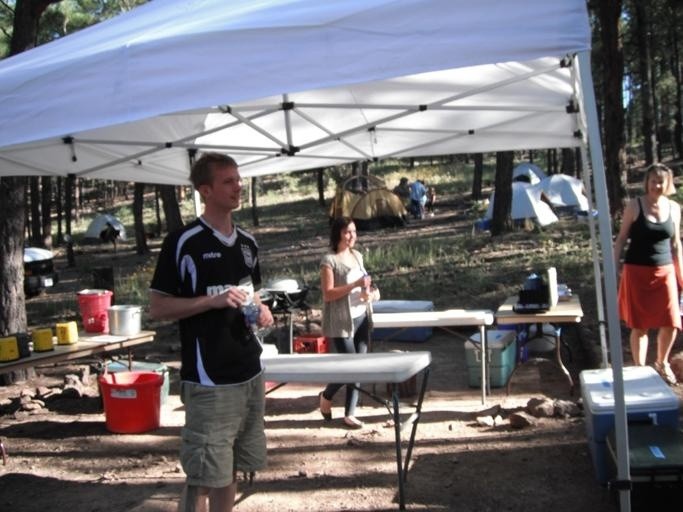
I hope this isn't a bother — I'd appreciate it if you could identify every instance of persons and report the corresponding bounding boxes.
[392,177,437,222]
[145,152,278,512]
[474,195,490,220]
[616,160,682,391]
[104,222,117,249]
[455,197,477,218]
[317,214,382,429]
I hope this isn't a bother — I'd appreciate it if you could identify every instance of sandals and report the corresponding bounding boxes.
[655,359,679,385]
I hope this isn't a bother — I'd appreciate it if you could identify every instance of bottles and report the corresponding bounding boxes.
[239,292,271,338]
[361,274,370,304]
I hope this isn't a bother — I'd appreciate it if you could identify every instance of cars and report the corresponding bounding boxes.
[22,257,59,299]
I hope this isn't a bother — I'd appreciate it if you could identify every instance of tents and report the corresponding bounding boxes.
[512,160,546,185]
[326,173,410,233]
[474,182,561,233]
[84,215,126,240]
[533,171,598,220]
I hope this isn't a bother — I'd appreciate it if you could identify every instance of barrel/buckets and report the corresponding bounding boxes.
[105,304,142,336]
[79,290,113,332]
[97,371,164,433]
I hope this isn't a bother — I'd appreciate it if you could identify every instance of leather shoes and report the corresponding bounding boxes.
[318,390,336,422]
[345,417,367,430]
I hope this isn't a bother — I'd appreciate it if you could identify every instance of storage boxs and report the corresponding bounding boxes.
[372,299,433,342]
[463,330,517,388]
[578,365,683,512]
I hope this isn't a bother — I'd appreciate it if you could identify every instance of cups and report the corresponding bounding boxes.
[0,321,79,363]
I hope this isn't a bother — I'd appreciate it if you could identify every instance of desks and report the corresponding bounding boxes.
[371,309,494,406]
[244,351,432,512]
[0,331,156,374]
[494,293,584,395]
